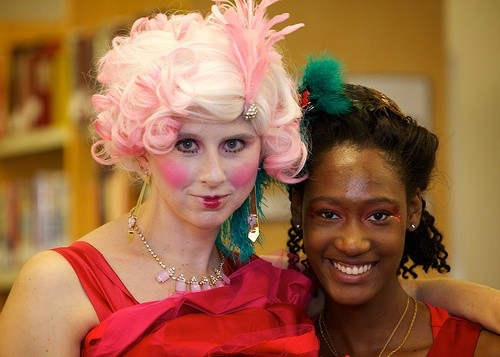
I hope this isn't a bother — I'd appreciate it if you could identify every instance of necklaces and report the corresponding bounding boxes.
[128,207,231,293]
[316,292,419,356]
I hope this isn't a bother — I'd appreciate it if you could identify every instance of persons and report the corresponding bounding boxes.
[286,55,500,357]
[0,0,500,356]
